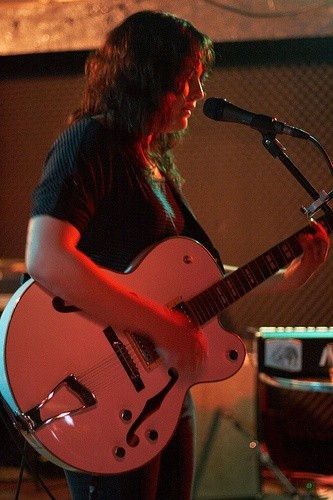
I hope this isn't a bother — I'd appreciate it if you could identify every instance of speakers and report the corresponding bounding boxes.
[253,328,333,499]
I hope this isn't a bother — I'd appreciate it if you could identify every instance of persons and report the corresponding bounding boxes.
[24,8,331,500]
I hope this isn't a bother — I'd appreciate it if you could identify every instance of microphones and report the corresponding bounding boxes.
[202,97,311,139]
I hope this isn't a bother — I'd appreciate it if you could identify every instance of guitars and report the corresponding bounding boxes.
[0,207,333,479]
